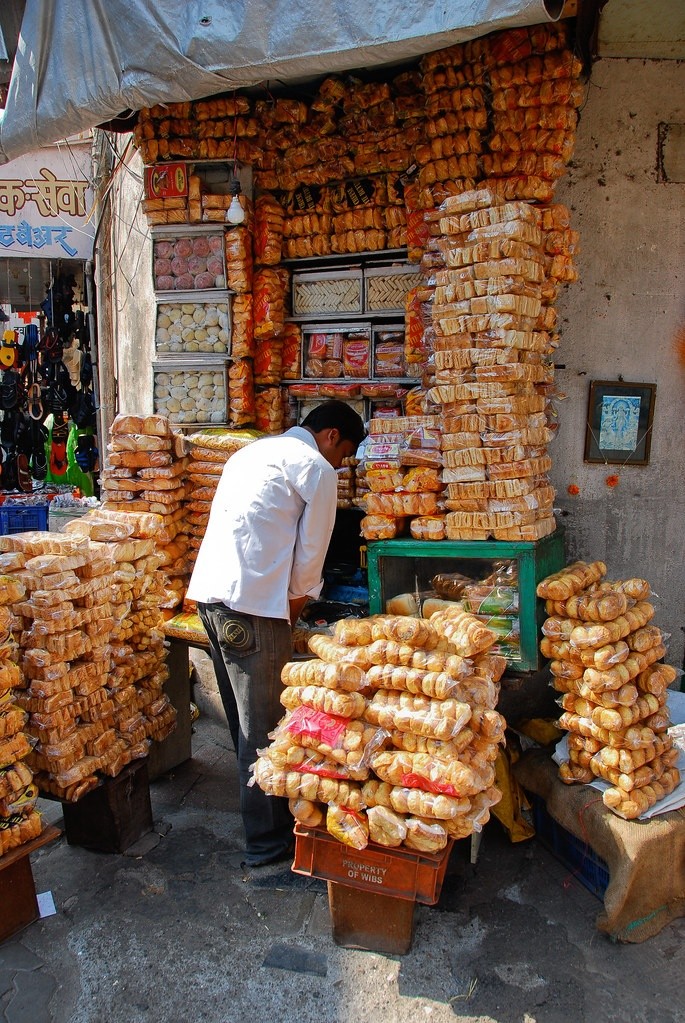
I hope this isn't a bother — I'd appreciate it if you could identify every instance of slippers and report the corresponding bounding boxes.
[0,265,98,492]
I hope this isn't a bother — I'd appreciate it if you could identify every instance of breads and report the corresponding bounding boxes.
[0,20,681,859]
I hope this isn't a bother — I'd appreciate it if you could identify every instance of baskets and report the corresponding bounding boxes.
[0,499,50,536]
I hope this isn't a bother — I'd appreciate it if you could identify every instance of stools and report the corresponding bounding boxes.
[0,823,68,947]
[35,752,164,873]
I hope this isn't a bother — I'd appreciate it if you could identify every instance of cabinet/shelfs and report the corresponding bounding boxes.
[364,532,566,674]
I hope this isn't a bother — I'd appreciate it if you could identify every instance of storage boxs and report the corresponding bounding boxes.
[535,794,621,900]
[141,162,190,198]
[48,497,92,532]
[280,818,459,907]
[1,498,51,535]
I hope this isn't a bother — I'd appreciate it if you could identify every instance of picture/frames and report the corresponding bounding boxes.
[584,379,657,466]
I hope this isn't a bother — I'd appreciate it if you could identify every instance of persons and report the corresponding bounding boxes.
[183,396,366,866]
